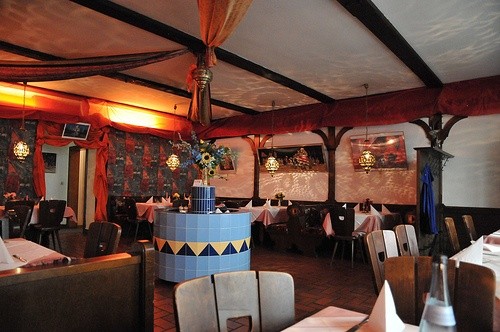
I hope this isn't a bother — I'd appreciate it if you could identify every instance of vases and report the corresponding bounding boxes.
[277,200,281,207]
[201,167,210,185]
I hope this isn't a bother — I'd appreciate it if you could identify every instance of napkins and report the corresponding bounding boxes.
[367,278,405,332]
[458,235,484,265]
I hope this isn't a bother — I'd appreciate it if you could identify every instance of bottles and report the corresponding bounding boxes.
[417,254,458,332]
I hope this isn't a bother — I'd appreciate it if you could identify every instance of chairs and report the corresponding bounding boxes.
[172,269,296,332]
[327,204,496,332]
[4,194,152,257]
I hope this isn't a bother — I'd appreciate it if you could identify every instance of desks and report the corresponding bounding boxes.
[0,238,71,270]
[239,204,289,247]
[280,303,420,332]
[452,229,500,332]
[135,203,171,238]
[321,211,401,264]
[0,205,79,239]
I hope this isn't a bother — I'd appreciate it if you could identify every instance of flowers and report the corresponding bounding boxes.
[275,192,285,201]
[170,130,232,182]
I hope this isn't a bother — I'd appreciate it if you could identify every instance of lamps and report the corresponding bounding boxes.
[358,82,378,176]
[165,104,180,172]
[13,80,30,164]
[264,100,280,178]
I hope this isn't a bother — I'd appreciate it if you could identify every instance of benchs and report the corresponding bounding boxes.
[268,203,324,257]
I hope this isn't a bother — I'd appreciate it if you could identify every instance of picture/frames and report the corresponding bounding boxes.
[216,132,408,174]
[42,123,91,173]
